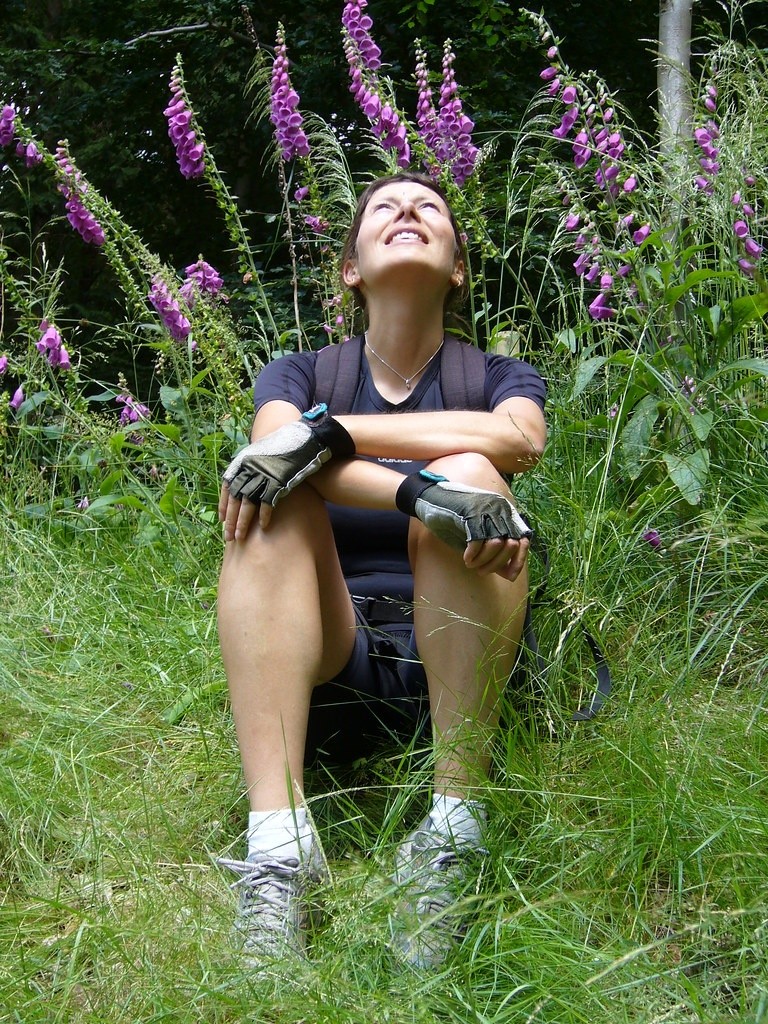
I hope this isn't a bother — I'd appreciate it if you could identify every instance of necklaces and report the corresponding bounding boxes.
[364,330,443,390]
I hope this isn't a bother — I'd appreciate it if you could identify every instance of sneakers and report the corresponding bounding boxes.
[213,844,326,984]
[393,819,487,970]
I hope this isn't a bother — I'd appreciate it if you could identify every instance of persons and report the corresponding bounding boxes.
[214,171,548,977]
